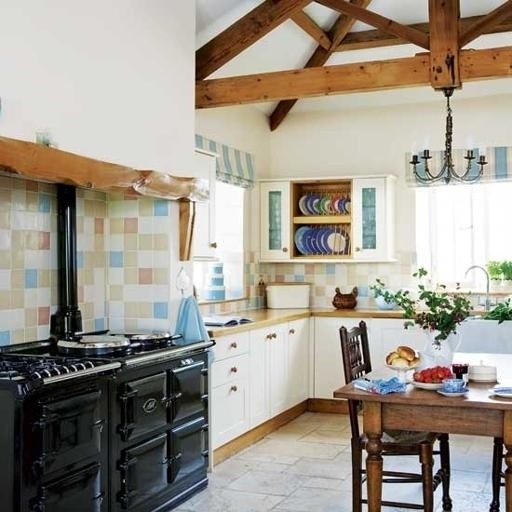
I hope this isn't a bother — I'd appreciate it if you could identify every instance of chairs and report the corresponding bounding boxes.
[339,320,454,511]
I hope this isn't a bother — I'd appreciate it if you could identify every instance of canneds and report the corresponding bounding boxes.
[452,364,468,385]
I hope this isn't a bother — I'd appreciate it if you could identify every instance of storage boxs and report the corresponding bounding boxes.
[266,282,311,309]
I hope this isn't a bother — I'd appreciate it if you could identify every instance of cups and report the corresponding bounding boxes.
[441,378,466,393]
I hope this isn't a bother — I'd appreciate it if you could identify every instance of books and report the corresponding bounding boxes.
[200,315,253,327]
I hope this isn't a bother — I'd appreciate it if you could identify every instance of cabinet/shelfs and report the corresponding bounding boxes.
[251,323,290,425]
[258,177,396,263]
[210,332,252,451]
[118,360,210,511]
[289,317,310,408]
[24,388,109,512]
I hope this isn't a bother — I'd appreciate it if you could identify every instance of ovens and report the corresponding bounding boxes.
[14,351,207,512]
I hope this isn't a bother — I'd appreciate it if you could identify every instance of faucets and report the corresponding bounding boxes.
[465,266,490,297]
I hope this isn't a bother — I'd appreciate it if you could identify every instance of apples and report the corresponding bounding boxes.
[414,366,453,383]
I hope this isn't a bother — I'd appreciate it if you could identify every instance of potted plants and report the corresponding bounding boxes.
[368,267,474,367]
[485,259,511,291]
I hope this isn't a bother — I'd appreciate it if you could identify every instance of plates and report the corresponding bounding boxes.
[295,226,349,255]
[489,387,512,398]
[298,195,352,216]
[436,388,469,398]
[411,379,465,391]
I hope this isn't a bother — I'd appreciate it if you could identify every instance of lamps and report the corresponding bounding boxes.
[409,88,487,187]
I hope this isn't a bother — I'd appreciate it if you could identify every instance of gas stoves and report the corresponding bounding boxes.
[0,330,216,384]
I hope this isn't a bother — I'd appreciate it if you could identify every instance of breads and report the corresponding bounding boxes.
[386,346,419,366]
